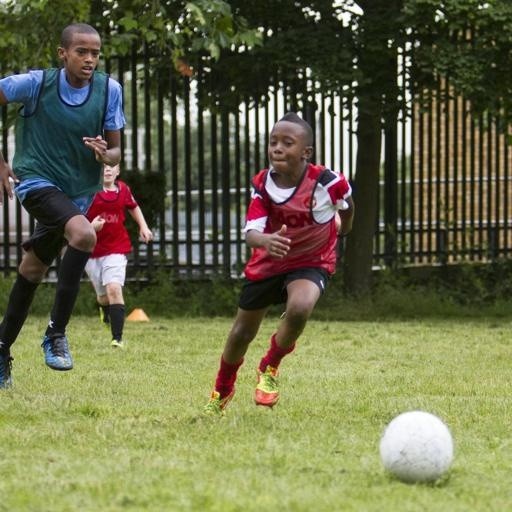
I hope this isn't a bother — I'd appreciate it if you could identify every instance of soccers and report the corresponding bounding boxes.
[380,411,453,481]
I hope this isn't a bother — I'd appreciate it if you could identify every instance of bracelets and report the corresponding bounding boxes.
[338,232,351,237]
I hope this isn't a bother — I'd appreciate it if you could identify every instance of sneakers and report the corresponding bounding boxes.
[42,333,73,370]
[204,389,233,416]
[255,364,279,406]
[98,307,109,326]
[0,340,13,391]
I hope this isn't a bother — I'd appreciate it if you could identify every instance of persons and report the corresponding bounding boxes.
[206,110,357,413]
[81,155,156,350]
[0,24,126,393]
